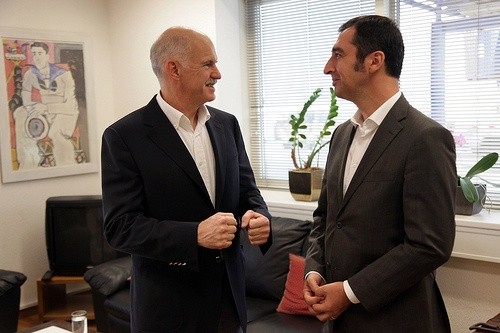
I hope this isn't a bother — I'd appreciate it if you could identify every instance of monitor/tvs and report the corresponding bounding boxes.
[47,196,131,278]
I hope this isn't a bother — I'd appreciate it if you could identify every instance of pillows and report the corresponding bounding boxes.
[275,252,315,315]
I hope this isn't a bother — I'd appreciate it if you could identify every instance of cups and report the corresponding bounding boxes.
[71,310,88,333]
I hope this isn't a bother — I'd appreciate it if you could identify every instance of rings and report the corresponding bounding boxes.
[331,316,336,320]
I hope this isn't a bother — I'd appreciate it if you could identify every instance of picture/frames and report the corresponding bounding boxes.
[0,28,100,182]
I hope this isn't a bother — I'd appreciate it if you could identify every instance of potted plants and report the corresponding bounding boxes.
[287,87,339,201]
[456,152,499,216]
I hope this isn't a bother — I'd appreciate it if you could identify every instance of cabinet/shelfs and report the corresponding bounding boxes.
[37,278,95,324]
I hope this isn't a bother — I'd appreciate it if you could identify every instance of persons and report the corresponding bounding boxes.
[101,26,273,333]
[302,15,458,333]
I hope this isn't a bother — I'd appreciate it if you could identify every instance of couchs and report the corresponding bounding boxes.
[84,216,325,333]
